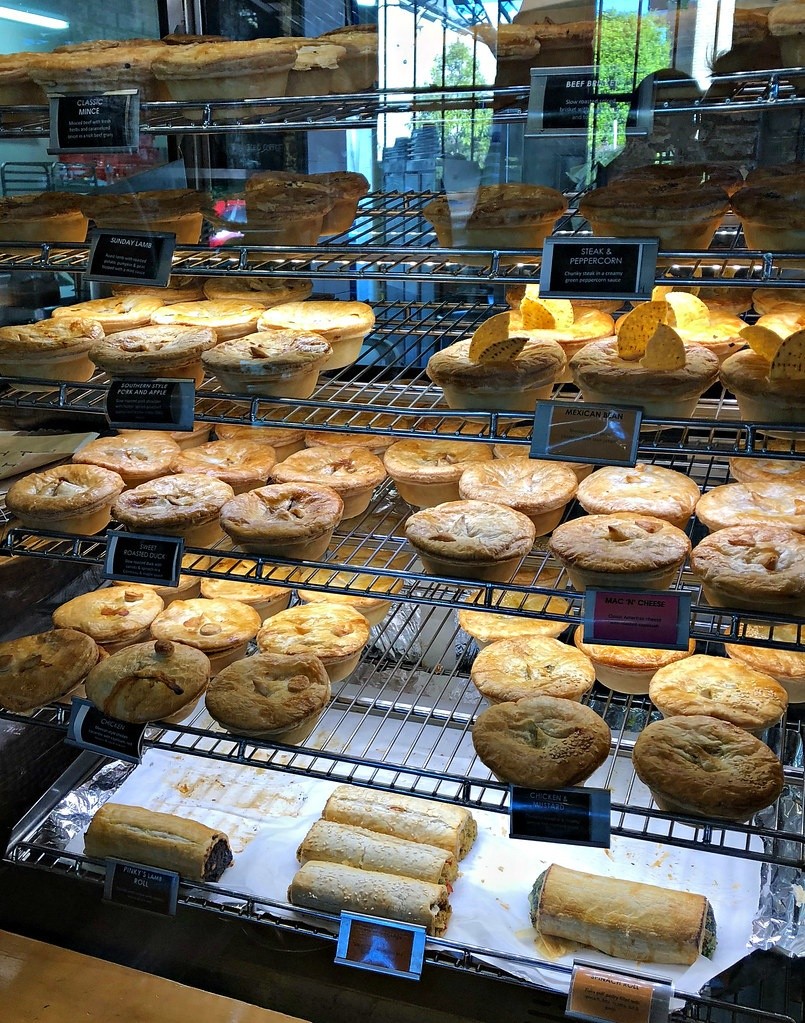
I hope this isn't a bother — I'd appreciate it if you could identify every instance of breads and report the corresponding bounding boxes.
[81,783,718,966]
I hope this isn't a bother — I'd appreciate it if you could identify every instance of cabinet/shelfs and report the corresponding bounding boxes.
[1,70,804,1023]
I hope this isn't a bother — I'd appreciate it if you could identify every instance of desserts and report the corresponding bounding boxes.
[0,0,805,830]
